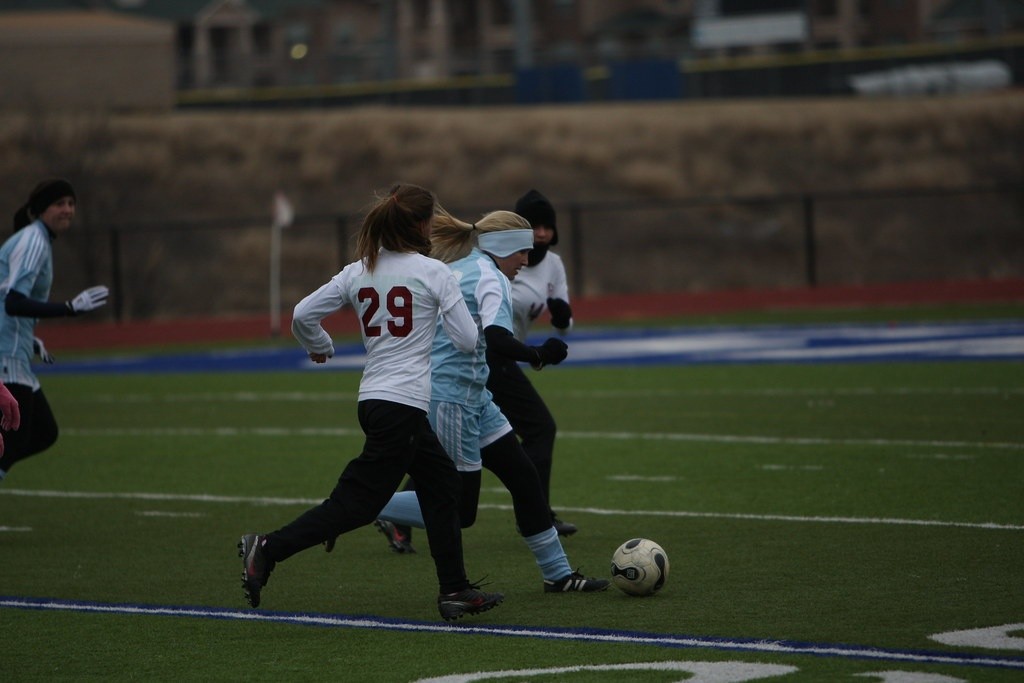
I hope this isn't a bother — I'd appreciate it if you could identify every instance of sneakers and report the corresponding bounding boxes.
[237,533,274,608]
[551,518,577,537]
[326,534,338,553]
[379,518,416,555]
[542,573,610,594]
[438,587,505,624]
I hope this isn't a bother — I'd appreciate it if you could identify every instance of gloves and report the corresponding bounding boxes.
[1,383,21,432]
[530,338,569,370]
[30,338,55,365]
[546,296,573,328]
[66,286,109,316]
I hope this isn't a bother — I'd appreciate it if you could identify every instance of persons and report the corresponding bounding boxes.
[216,10,956,116]
[373,181,581,556]
[1,166,109,477]
[322,207,613,597]
[237,184,508,620]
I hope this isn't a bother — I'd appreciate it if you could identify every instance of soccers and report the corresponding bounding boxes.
[608,539,668,596]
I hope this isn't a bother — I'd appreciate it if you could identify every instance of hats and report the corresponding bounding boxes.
[515,190,559,247]
